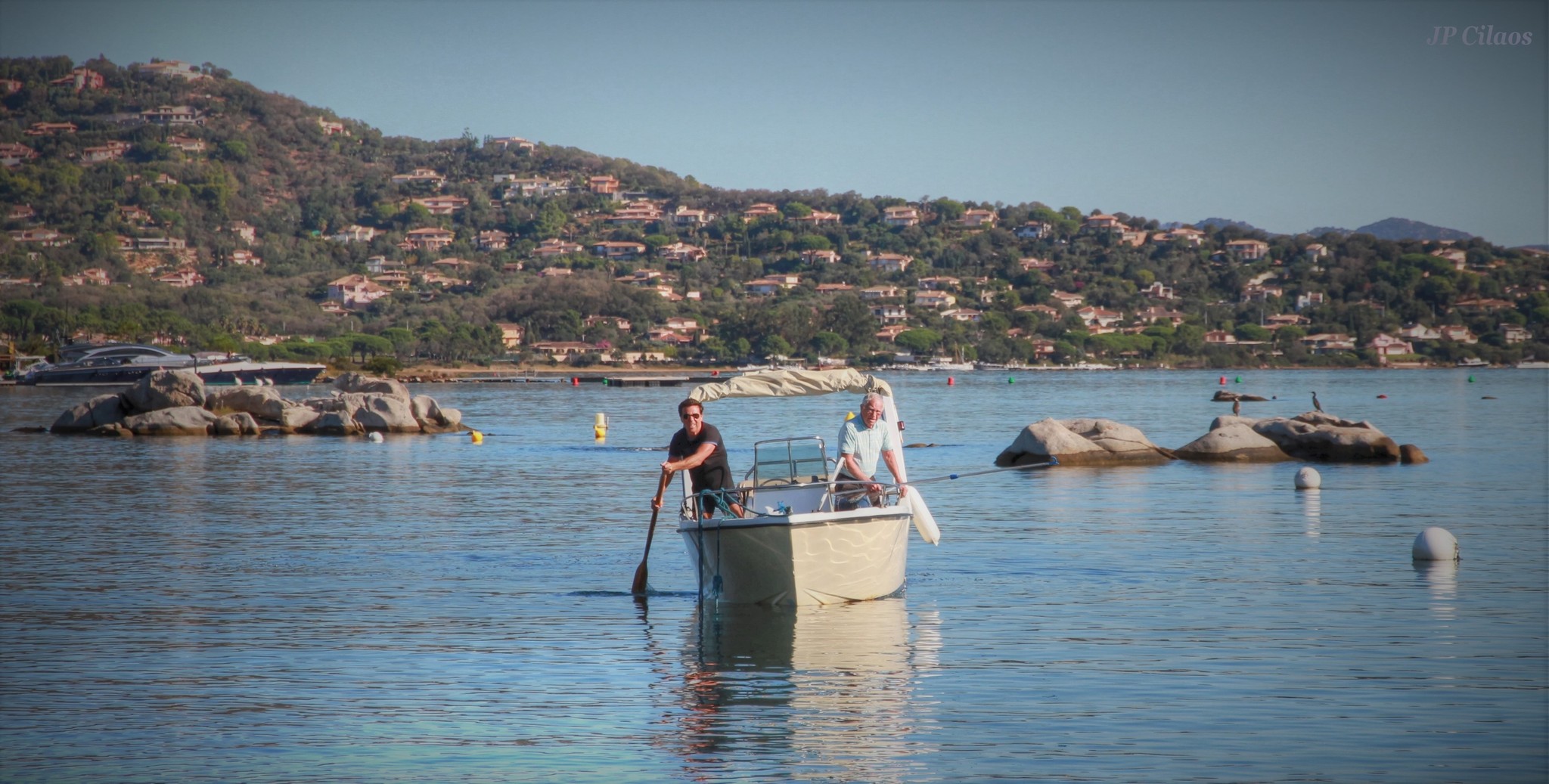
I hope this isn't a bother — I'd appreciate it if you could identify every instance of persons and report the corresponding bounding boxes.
[650,398,745,520]
[835,393,907,507]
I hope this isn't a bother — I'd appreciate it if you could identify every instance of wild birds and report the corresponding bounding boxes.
[1309,391,1326,413]
[1232,395,1240,416]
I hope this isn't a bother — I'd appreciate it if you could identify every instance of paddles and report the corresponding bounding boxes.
[630,472,667,593]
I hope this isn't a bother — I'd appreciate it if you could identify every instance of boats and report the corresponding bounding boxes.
[675,436,911,608]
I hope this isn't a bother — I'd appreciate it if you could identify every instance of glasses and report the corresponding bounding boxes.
[864,405,881,415]
[683,413,700,420]
